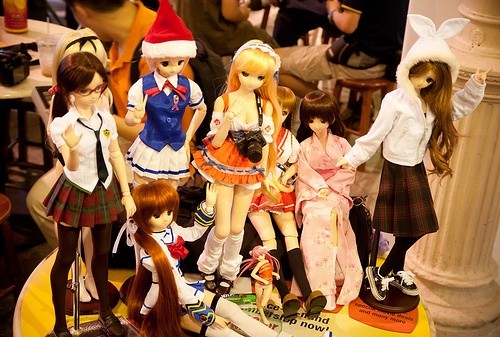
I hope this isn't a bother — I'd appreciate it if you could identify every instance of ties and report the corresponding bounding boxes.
[77,113,109,183]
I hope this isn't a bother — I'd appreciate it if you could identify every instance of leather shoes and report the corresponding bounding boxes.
[202,269,218,292]
[304,291,327,320]
[97,313,123,335]
[282,293,300,321]
[218,276,234,295]
[53,327,73,337]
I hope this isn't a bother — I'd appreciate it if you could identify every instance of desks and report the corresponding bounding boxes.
[0,16,76,192]
[13,248,438,337]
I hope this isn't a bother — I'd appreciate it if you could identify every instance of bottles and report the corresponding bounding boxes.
[2,0,29,33]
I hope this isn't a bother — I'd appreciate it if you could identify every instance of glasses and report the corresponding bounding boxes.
[71,81,108,97]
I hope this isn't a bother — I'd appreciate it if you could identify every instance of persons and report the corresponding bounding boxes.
[40,52,140,337]
[294,90,366,314]
[172,0,281,57]
[191,39,284,299]
[334,12,493,302]
[270,0,410,100]
[26,0,202,248]
[260,0,346,47]
[124,179,281,337]
[246,84,328,320]
[234,243,281,326]
[123,0,207,191]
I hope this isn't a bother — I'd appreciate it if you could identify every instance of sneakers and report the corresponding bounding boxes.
[388,274,419,296]
[365,266,386,301]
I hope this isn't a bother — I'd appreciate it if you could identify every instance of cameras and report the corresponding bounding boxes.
[235,129,266,163]
[0,43,32,86]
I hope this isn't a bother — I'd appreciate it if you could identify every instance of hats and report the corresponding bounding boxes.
[141,0,197,59]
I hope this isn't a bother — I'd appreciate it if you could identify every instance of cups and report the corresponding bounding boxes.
[34,34,61,76]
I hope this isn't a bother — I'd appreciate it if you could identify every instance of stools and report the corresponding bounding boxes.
[334,79,392,137]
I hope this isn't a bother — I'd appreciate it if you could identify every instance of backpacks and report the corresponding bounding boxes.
[129,31,228,153]
